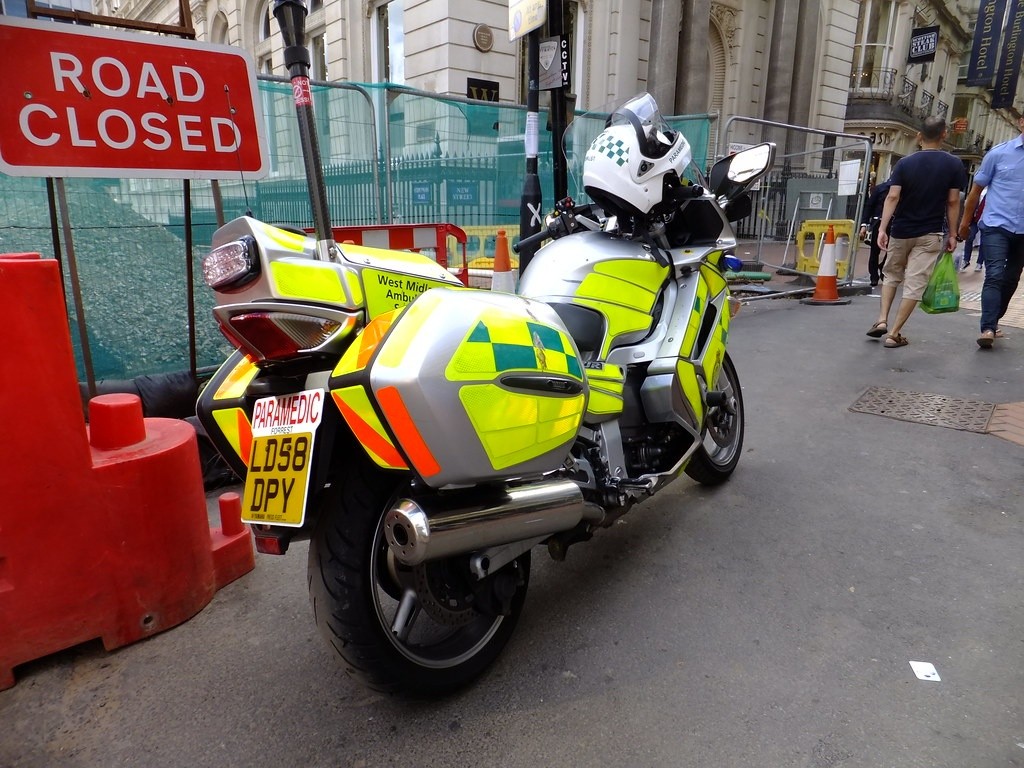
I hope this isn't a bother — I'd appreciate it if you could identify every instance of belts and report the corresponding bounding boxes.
[874,217,882,220]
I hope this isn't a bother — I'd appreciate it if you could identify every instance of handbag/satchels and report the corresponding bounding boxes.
[917,250,960,315]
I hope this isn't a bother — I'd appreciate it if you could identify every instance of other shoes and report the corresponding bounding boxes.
[976,331,995,349]
[878,264,884,282]
[974,263,983,272]
[993,328,1005,339]
[869,280,879,287]
[959,261,970,271]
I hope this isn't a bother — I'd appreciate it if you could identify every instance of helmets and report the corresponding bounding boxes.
[582,126,691,215]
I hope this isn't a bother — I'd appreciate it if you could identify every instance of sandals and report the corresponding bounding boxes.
[865,320,888,338]
[883,332,909,347]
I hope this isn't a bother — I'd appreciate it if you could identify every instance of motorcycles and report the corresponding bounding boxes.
[192,0,779,703]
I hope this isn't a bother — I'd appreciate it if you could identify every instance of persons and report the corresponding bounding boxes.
[859,116,968,347]
[958,110,1024,348]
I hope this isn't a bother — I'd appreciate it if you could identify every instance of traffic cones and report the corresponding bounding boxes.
[798,225,853,306]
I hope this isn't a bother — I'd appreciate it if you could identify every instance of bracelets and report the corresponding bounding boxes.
[860,226,866,228]
[950,233,957,239]
